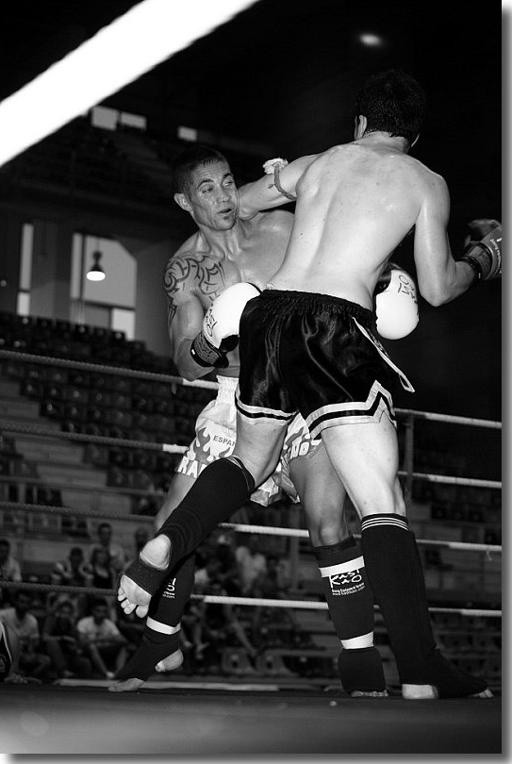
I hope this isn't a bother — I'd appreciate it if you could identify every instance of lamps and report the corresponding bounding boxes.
[86,228,105,281]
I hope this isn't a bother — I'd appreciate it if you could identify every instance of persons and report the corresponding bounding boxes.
[117,69,503,700]
[109,143,419,706]
[1,309,500,698]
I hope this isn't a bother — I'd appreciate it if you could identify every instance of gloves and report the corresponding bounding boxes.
[456,219,502,280]
[190,282,262,368]
[372,262,420,340]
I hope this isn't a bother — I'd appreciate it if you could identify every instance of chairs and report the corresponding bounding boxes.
[1,311,501,701]
